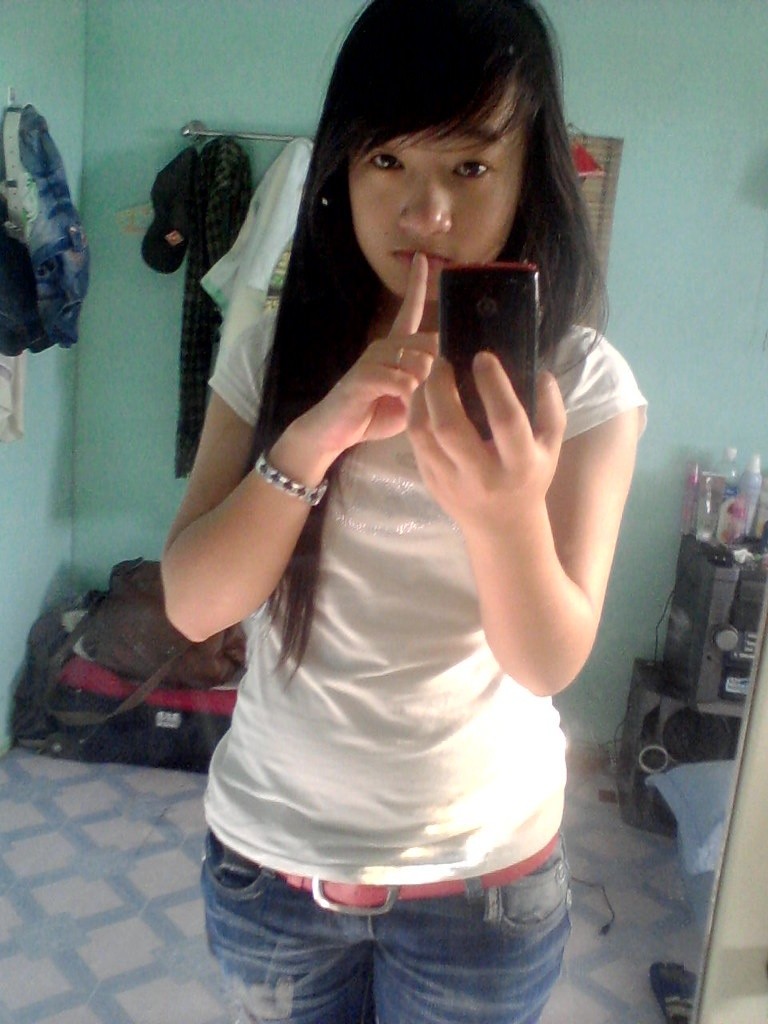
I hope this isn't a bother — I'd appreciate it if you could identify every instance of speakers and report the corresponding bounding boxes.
[612,535,768,836]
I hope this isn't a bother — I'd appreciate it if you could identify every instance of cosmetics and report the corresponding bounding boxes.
[681,444,768,549]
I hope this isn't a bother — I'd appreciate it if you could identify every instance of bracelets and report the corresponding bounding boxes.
[256,451,327,506]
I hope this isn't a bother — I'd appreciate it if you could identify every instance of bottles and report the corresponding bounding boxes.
[714,448,768,545]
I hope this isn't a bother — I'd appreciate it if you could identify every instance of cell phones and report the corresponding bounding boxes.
[438,260,541,443]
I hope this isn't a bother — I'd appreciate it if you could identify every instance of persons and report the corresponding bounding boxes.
[159,0,649,1024]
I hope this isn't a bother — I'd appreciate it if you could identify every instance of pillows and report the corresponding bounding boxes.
[643,759,737,875]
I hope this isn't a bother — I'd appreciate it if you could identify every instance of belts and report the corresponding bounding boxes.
[253,831,561,916]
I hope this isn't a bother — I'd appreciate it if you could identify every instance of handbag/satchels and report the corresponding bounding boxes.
[39,558,247,728]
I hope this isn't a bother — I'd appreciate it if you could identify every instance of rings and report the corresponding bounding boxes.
[395,347,405,366]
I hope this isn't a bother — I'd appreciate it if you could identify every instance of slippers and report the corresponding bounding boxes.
[650,960,694,1024]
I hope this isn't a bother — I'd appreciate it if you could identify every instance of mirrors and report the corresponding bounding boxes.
[0,0,768,1024]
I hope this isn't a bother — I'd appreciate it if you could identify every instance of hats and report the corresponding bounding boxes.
[141,145,198,274]
